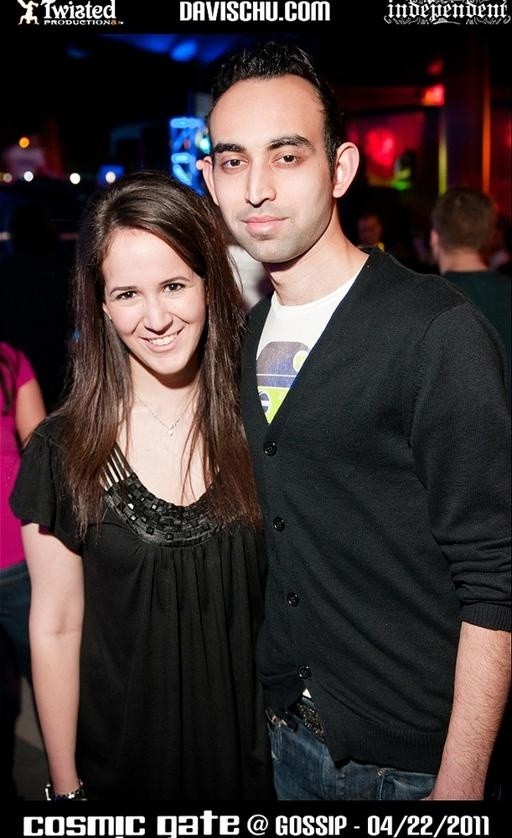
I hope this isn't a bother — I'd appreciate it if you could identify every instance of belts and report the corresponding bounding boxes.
[289,699,325,741]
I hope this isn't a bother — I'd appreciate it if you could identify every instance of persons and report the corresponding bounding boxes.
[193,38,510,804]
[12,178,278,811]
[343,188,511,363]
[0,339,48,748]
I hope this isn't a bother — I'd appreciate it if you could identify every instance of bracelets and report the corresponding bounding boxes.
[42,778,84,801]
